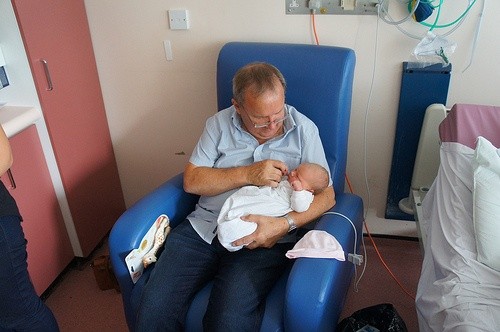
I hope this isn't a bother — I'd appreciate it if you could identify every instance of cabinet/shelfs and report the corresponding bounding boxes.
[0,0,126,298]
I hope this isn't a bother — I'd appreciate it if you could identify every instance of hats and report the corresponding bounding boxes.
[285,230,346,261]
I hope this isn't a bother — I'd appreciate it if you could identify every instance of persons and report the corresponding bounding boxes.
[216,162,329,252]
[134,61,335,332]
[0,125,59,332]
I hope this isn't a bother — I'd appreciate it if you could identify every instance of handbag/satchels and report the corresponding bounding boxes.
[89,258,113,290]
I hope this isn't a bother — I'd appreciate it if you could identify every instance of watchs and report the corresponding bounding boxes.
[283,213,297,236]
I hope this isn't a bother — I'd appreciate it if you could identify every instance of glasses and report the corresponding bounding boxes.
[232,96,288,129]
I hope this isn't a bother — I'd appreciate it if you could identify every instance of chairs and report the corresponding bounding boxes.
[108,41,363,332]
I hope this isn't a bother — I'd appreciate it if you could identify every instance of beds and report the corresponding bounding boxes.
[399,104,500,332]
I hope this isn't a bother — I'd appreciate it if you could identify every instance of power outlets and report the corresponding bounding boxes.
[286,0,390,15]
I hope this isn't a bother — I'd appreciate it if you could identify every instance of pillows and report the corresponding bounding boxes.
[474,137,500,273]
